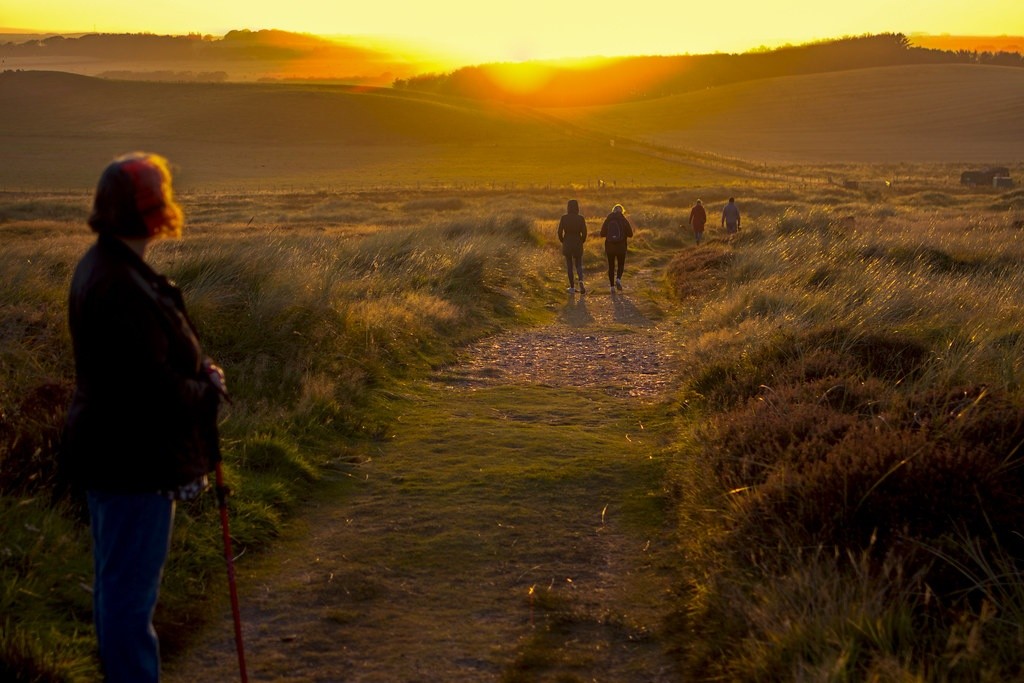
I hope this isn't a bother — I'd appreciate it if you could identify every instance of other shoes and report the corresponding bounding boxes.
[611,287,615,292]
[579,281,585,294]
[567,287,575,293]
[616,279,622,290]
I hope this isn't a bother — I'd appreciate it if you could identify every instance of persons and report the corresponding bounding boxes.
[689,199,707,245]
[558,200,586,295]
[67,153,231,683]
[722,197,741,236]
[601,205,634,293]
